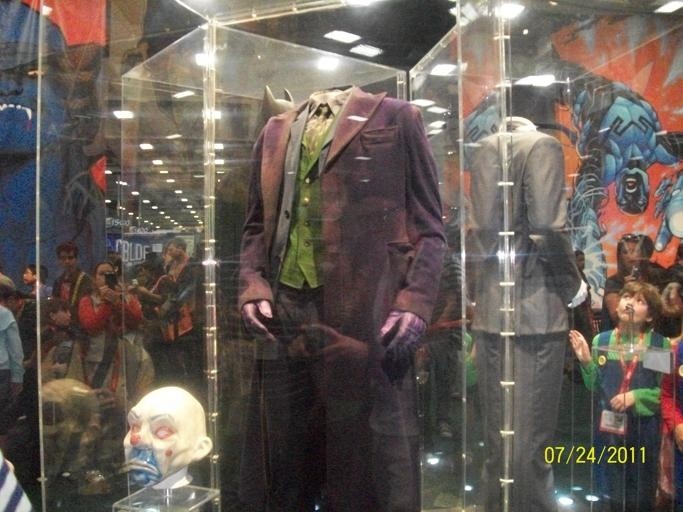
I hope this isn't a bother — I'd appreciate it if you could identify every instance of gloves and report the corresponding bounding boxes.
[378,308,429,365]
[239,297,277,344]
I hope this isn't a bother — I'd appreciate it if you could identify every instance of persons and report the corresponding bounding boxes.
[469,73,593,511]
[569,233,682,512]
[236,85,449,512]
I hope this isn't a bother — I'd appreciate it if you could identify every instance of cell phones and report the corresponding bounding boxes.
[105,271,119,290]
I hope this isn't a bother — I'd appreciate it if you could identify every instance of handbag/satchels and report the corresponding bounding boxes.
[154,273,195,346]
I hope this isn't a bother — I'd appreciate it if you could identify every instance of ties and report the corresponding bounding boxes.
[306,104,331,160]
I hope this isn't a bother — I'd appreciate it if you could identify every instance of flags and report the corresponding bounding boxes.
[89,156,107,195]
